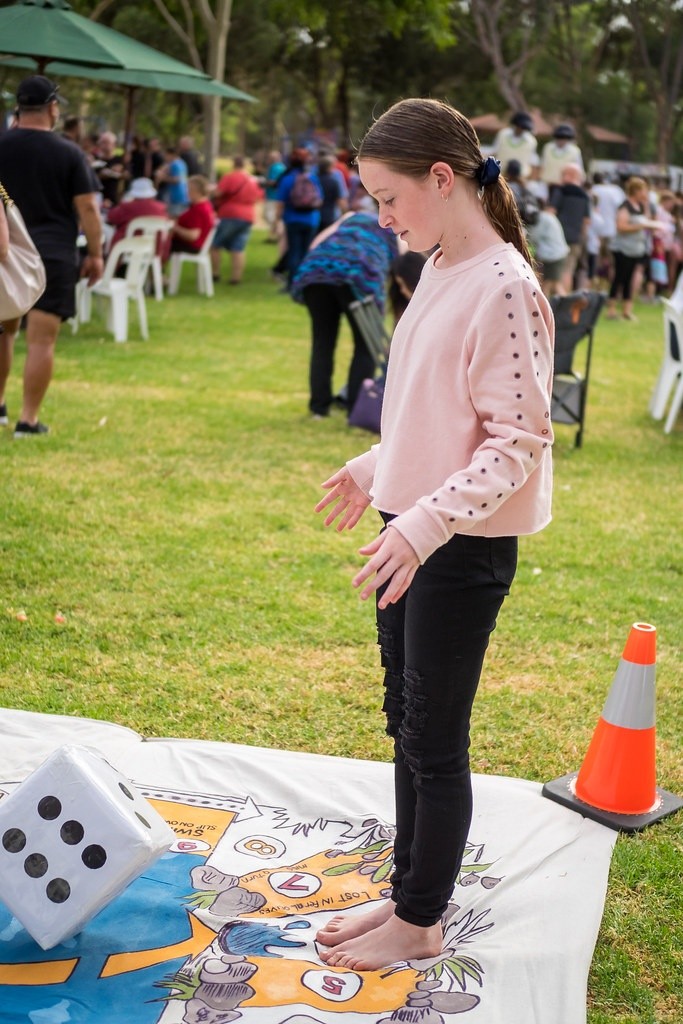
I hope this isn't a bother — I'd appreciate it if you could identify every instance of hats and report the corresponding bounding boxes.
[507,159,522,177]
[552,125,577,140]
[510,112,534,132]
[15,76,70,107]
[289,148,308,162]
[127,177,158,200]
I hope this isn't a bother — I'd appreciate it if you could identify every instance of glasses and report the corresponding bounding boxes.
[44,83,60,104]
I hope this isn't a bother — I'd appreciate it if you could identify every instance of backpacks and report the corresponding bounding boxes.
[288,165,325,211]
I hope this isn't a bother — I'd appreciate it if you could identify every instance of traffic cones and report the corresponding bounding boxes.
[542,621,683,836]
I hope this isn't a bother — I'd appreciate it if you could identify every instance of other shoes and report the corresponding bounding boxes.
[13,421,50,438]
[309,398,330,419]
[0,405,9,425]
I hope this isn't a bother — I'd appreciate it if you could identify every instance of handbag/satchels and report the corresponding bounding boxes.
[0,183,47,322]
[210,191,236,214]
[348,362,387,437]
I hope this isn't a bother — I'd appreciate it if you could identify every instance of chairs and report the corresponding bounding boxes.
[78,216,222,343]
[646,298,683,432]
[351,296,393,371]
[545,289,605,446]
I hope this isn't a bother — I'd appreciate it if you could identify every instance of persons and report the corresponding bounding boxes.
[313,97,555,968]
[0,75,105,436]
[60,116,216,272]
[212,155,261,285]
[491,111,683,320]
[262,148,437,324]
[289,211,398,419]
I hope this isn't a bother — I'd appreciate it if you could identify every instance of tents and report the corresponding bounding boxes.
[0,0,261,203]
[465,106,629,151]
